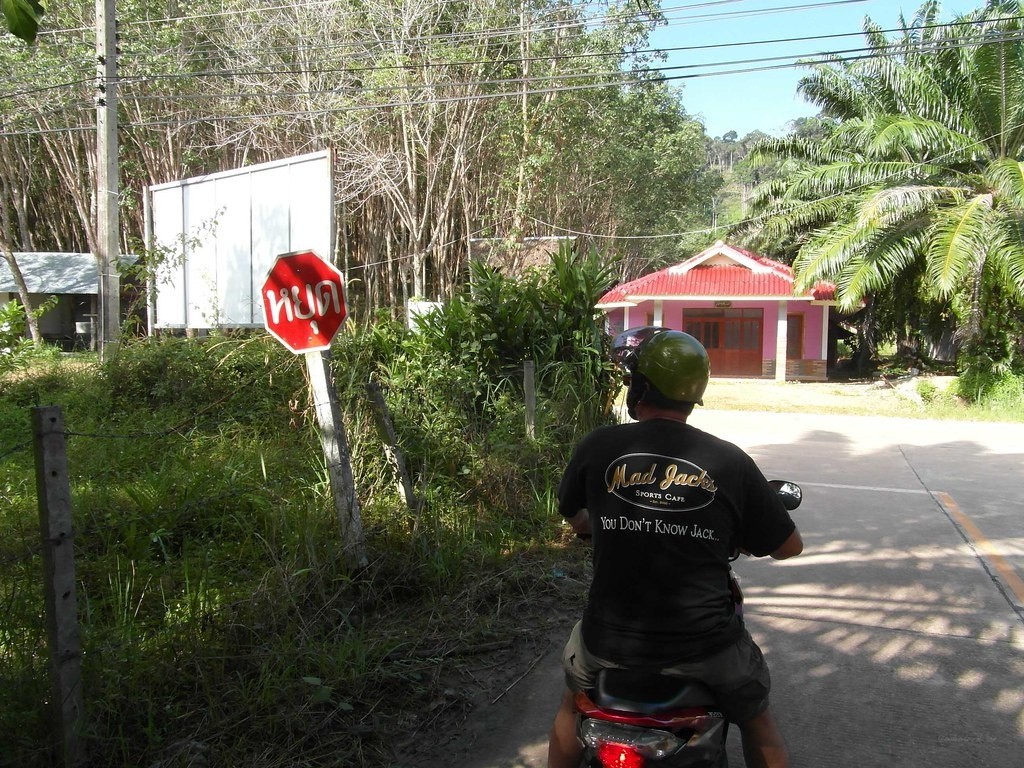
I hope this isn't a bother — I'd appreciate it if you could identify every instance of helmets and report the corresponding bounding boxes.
[626,330,710,404]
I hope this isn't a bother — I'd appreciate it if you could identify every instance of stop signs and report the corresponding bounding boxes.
[260,249,349,356]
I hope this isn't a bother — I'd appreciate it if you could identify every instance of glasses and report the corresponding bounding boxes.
[623,374,634,386]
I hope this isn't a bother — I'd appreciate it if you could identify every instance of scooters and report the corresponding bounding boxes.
[560,480,802,768]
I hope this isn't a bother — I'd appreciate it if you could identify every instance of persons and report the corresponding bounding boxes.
[548,325,803,768]
[770,482,798,509]
